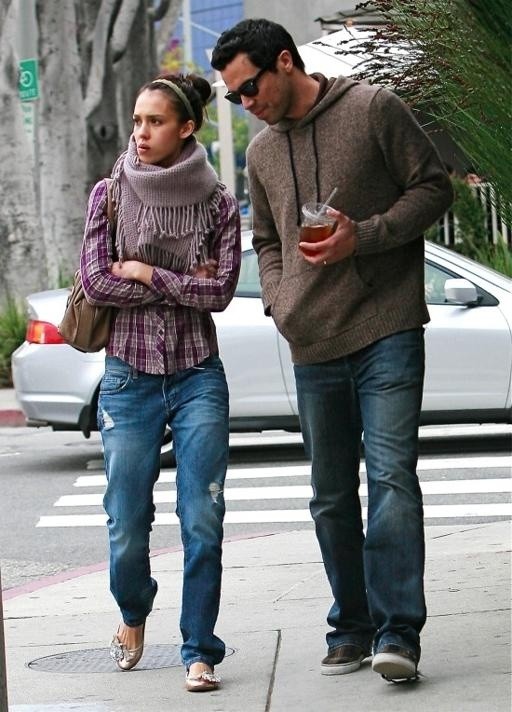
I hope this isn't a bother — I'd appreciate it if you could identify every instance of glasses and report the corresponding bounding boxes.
[223,61,272,108]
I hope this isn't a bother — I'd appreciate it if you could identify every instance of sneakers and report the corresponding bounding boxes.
[371,641,418,679]
[319,645,366,676]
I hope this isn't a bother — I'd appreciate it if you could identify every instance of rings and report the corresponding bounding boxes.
[323,260,328,265]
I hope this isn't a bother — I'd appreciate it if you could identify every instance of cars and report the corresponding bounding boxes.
[11,230,512,467]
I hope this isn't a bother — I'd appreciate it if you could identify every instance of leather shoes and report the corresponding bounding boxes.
[184,668,217,692]
[112,615,146,670]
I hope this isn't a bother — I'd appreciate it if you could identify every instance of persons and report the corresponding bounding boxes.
[424,282,435,300]
[81,74,244,695]
[439,153,512,249]
[214,15,455,682]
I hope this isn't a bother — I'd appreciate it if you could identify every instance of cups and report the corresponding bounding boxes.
[298,202,338,257]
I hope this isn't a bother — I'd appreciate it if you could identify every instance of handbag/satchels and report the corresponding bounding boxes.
[58,265,116,356]
